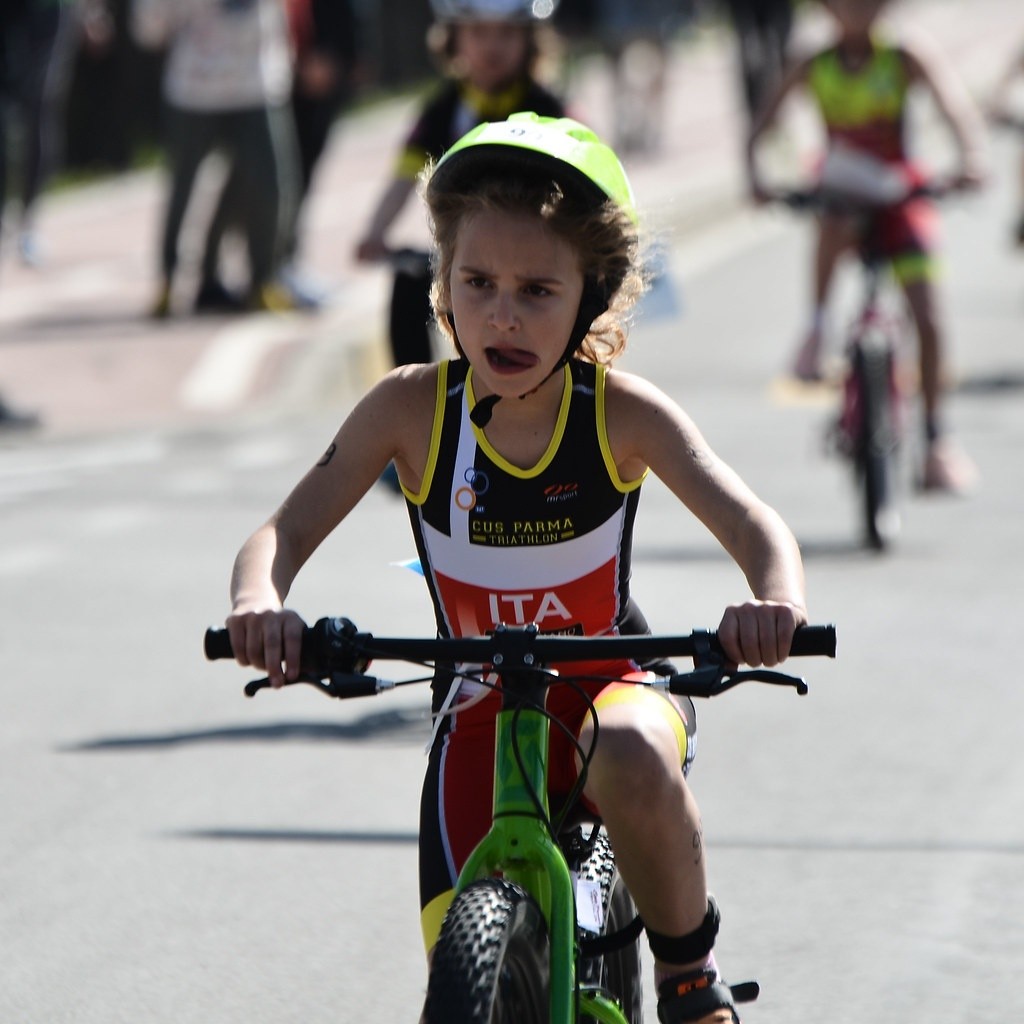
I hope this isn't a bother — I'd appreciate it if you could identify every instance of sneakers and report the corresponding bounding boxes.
[654,968,740,1024]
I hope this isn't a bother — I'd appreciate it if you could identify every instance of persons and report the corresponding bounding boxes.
[746,0,982,493]
[1,0,113,430]
[598,0,694,168]
[192,0,381,310]
[355,0,572,261]
[132,0,326,302]
[729,0,816,209]
[226,114,812,1021]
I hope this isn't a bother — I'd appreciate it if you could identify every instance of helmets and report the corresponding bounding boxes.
[424,110,640,249]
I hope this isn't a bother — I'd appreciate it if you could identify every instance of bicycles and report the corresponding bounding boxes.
[197,611,842,1024]
[366,240,446,503]
[750,168,983,557]
